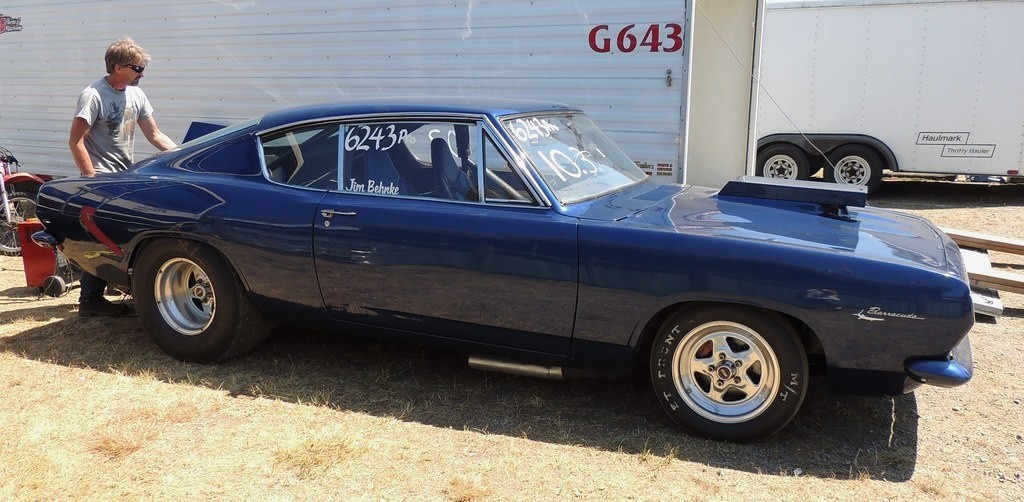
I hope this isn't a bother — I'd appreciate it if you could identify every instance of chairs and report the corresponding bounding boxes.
[430,138,478,203]
[360,145,420,198]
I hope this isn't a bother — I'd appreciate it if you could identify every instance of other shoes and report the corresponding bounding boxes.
[78,297,126,316]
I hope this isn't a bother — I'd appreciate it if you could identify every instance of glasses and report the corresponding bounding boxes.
[122,63,145,72]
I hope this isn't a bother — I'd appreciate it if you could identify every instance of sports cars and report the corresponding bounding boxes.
[30,98,977,444]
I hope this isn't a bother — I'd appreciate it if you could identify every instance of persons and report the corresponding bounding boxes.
[68,38,177,317]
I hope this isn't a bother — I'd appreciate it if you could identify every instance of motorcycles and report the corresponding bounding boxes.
[0,145,46,257]
